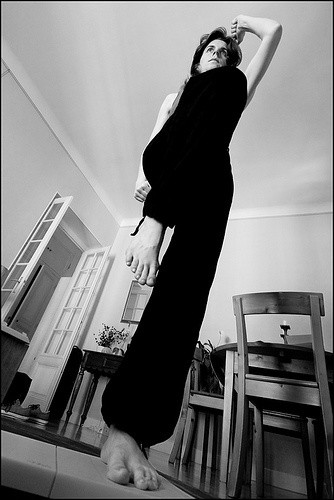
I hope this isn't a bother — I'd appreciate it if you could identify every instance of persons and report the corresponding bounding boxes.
[101,15,282,490]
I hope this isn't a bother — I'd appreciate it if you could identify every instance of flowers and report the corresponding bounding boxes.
[93,323,129,348]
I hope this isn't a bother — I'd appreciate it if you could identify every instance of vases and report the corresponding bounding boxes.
[96,346,111,354]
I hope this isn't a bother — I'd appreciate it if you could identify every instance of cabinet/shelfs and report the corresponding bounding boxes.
[1,227,72,339]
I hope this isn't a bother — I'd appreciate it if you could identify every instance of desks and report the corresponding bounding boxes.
[65,349,123,427]
[209,342,333,482]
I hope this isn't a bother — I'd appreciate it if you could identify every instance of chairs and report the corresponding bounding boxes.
[167,292,333,499]
